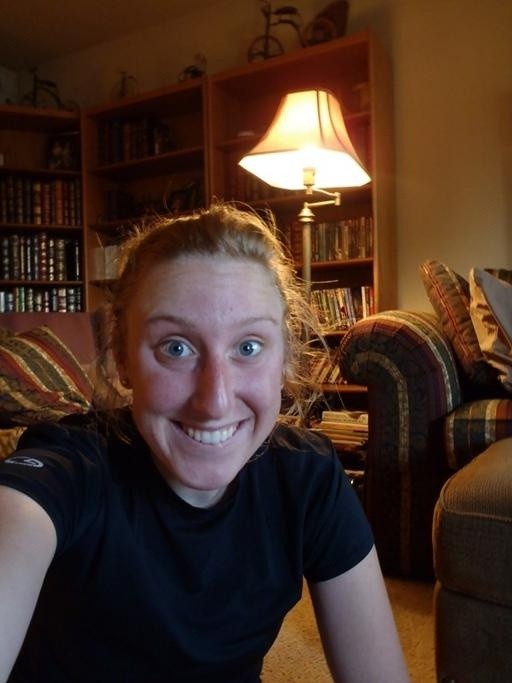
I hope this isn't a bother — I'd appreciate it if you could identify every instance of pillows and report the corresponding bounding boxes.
[0,325,99,429]
[418,257,512,396]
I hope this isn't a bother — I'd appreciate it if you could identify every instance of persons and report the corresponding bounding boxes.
[0,199,413,680]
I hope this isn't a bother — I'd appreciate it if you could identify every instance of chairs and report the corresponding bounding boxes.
[336,268,511,589]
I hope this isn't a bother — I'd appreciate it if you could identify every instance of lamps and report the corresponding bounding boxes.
[233,87,375,428]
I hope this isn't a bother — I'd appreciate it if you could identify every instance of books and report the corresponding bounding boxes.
[310,409,368,445]
[306,346,348,385]
[278,218,374,264]
[94,112,173,162]
[0,175,85,313]
[227,149,296,200]
[311,286,374,332]
